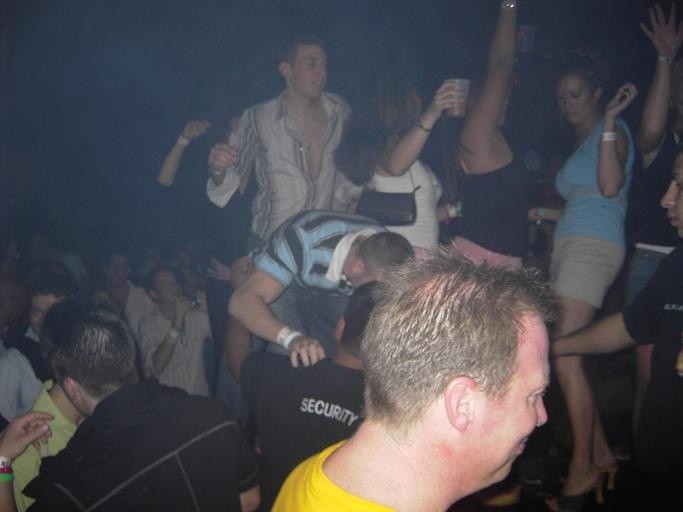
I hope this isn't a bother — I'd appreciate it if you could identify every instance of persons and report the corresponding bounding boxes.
[524,56,642,505]
[209,31,370,251]
[433,0,540,266]
[1,115,683,509]
[592,0,683,440]
[349,61,469,263]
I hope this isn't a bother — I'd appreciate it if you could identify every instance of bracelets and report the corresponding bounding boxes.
[654,53,677,66]
[499,1,523,10]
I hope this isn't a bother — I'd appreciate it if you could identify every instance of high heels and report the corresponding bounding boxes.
[596,446,621,490]
[557,466,604,511]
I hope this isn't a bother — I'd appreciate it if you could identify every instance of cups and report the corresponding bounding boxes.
[445,80,472,118]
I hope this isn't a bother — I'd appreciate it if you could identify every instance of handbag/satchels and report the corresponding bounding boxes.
[356,187,416,226]
